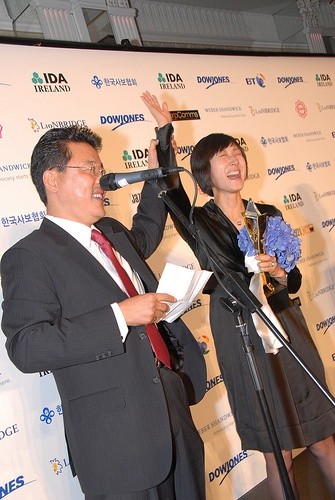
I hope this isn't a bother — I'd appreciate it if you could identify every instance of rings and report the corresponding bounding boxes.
[271,261,274,266]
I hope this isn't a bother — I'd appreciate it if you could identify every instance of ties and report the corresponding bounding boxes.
[90,228,173,370]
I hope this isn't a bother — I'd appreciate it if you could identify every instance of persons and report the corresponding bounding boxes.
[141,91,335,500]
[0,125,207,500]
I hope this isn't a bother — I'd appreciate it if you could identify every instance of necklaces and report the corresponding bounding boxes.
[214,200,244,225]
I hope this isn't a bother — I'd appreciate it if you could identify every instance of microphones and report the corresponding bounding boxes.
[100,166,184,191]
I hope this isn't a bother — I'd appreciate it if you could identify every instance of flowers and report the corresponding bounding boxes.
[237,216,301,273]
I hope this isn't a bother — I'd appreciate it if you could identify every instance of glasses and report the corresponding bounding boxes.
[50,165,107,177]
